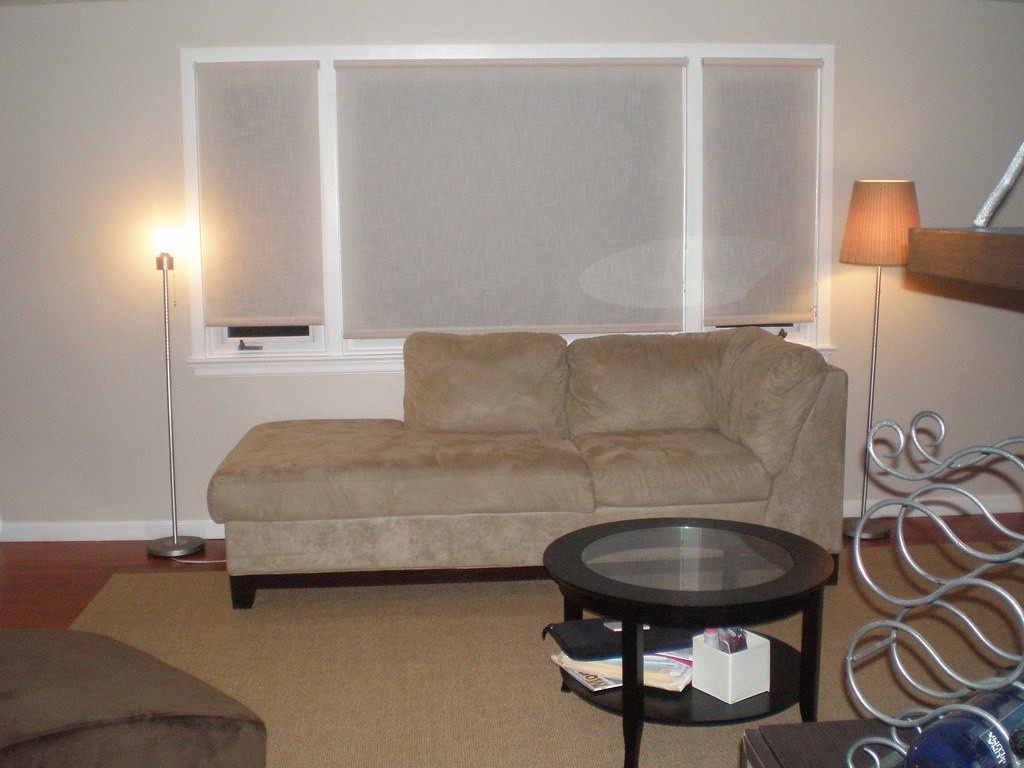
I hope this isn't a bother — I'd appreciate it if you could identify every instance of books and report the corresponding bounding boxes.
[548,620,698,693]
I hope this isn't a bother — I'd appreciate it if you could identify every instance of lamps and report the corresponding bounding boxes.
[839,179,922,539]
[145,228,206,559]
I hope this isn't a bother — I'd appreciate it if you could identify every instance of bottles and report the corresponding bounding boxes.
[704,627,719,650]
[905,665,1024,768]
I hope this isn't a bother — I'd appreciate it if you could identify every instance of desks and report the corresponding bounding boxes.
[739,713,1024,768]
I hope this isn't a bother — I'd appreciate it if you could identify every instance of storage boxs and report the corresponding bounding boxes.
[690,627,770,705]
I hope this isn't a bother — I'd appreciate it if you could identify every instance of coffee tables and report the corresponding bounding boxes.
[541,517,835,768]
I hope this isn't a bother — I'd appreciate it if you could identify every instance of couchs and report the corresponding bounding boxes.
[208,330,848,610]
[0,629,268,768]
